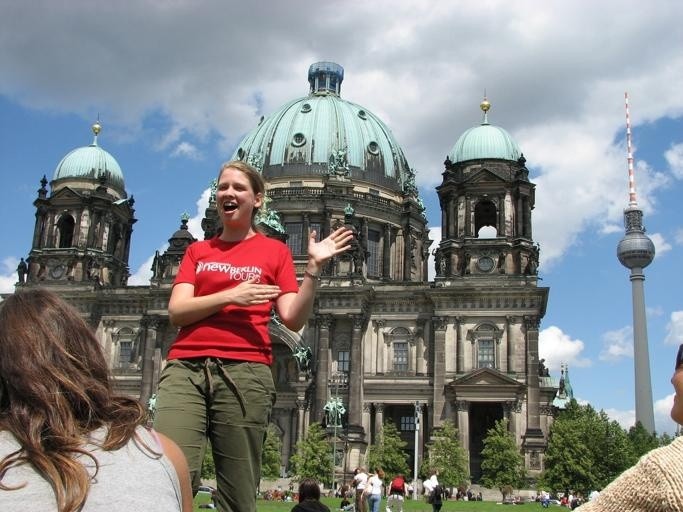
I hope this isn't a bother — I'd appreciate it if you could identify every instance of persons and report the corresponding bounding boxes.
[291,477,331,512]
[443,488,449,500]
[408,479,413,497]
[151,161,353,512]
[352,466,385,512]
[386,474,407,512]
[0,289,193,512]
[428,469,442,512]
[148,394,157,413]
[456,487,482,501]
[264,489,288,502]
[570,342,683,512]
[322,397,346,426]
[18,257,27,283]
[567,491,578,511]
[341,495,355,512]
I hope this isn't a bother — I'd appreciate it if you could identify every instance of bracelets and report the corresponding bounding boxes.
[305,269,320,280]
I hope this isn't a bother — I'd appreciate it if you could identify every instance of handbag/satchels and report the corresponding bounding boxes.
[364,485,372,495]
[353,479,362,487]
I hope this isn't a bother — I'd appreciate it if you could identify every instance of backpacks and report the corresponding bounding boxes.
[392,477,404,492]
